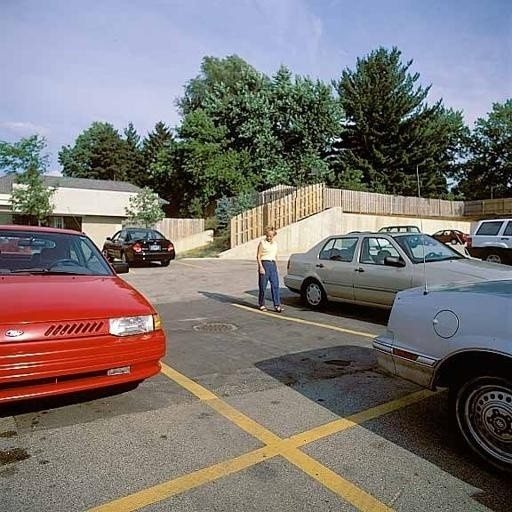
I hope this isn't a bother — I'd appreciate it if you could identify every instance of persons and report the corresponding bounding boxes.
[257,226,284,313]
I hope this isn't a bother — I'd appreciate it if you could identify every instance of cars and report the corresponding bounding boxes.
[371,277,509,478]
[432,230,470,245]
[0,223,175,403]
[285,231,509,311]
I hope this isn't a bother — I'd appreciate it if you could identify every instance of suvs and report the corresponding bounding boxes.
[466,220,510,264]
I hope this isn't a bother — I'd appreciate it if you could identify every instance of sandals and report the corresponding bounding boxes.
[260,306,268,312]
[274,305,281,313]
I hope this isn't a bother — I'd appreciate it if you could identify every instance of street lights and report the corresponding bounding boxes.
[416,158,434,198]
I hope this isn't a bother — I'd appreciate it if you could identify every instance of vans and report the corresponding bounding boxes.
[377,225,421,233]
